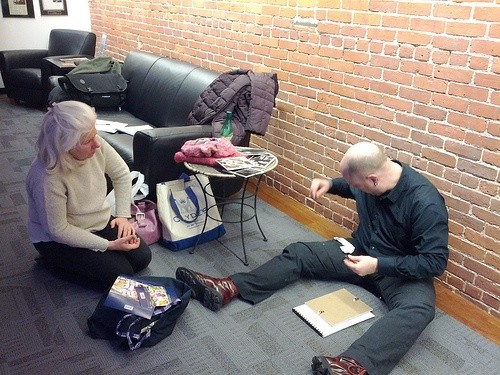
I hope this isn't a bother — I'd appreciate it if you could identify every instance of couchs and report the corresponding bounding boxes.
[48,51,252,207]
[0,29,96,112]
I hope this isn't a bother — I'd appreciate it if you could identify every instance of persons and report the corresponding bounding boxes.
[176,141,450,375]
[25,101,152,292]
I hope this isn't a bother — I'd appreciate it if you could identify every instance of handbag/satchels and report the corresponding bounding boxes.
[127,199,162,247]
[65,72,128,111]
[107,170,149,217]
[155,173,227,253]
[87,274,192,351]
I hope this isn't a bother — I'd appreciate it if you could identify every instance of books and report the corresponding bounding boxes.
[293,288,376,339]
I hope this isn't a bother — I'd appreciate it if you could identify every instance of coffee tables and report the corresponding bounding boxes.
[184,146,279,266]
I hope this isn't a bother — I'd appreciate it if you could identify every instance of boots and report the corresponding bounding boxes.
[176,267,237,312]
[311,356,369,375]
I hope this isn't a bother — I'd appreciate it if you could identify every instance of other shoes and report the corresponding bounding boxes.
[34,251,59,267]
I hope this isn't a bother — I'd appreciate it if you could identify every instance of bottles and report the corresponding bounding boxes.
[220,111,234,144]
